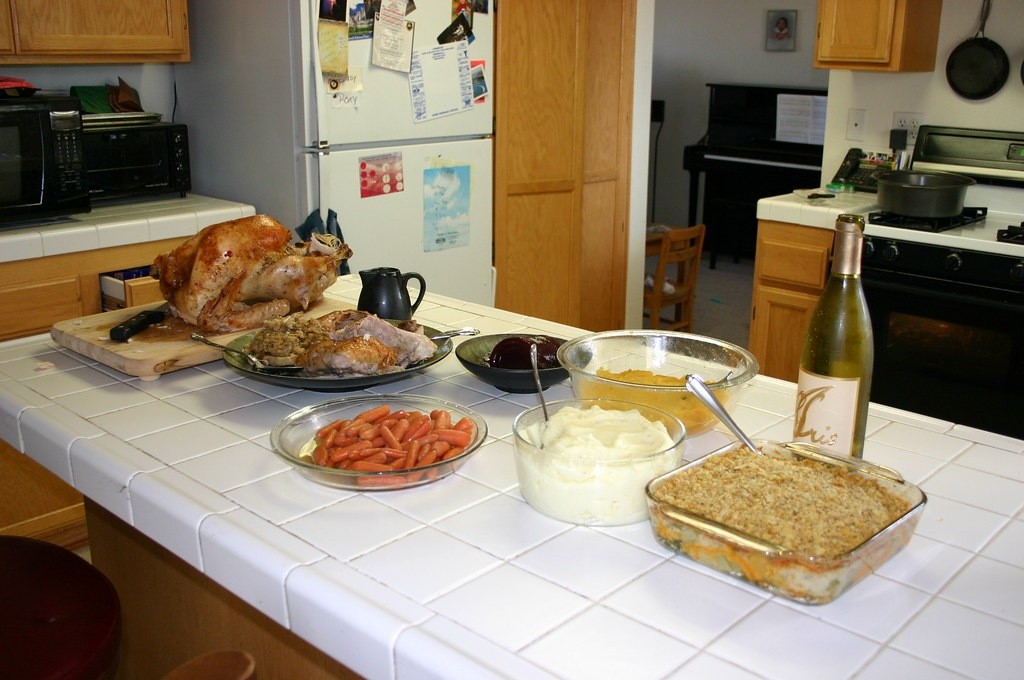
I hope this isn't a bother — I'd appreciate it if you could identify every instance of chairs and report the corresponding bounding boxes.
[645,224,706,334]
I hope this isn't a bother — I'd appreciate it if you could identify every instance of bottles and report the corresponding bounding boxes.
[790,214,874,466]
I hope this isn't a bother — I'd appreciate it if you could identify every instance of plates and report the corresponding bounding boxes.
[273,395,486,489]
[0,87,40,98]
[222,321,452,392]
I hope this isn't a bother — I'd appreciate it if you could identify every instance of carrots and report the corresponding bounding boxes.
[312,405,475,487]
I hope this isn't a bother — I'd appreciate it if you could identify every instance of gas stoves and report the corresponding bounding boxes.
[853,124,1024,258]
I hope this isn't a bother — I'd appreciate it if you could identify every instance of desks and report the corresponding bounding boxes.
[646,229,690,333]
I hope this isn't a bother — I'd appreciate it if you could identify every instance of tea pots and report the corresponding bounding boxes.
[355,267,425,322]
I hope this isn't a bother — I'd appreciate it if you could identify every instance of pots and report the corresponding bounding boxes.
[946,0,1010,100]
[870,169,977,217]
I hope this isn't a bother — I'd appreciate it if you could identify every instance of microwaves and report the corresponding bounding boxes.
[0,100,92,222]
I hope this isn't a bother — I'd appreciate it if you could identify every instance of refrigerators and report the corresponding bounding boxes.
[175,0,496,314]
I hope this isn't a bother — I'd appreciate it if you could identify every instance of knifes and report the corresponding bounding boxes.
[110,299,180,341]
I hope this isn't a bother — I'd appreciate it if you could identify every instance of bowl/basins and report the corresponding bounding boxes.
[643,438,929,606]
[456,333,590,395]
[514,398,687,527]
[558,330,761,442]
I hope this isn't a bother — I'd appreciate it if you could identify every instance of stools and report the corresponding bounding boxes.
[0,535,122,680]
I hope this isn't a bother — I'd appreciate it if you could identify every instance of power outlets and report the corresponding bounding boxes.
[892,111,926,146]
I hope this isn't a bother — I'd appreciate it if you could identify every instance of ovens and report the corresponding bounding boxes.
[845,235,1024,443]
[80,122,191,204]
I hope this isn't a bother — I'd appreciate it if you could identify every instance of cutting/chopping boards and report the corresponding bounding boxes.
[50,297,368,384]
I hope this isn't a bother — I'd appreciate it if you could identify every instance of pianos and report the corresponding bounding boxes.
[682,83,828,270]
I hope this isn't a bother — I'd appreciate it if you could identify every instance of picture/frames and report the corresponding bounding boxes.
[765,9,798,53]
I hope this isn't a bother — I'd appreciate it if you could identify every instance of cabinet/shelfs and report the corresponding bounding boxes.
[0,235,192,553]
[494,0,636,334]
[748,217,836,385]
[0,0,190,64]
[813,0,942,72]
